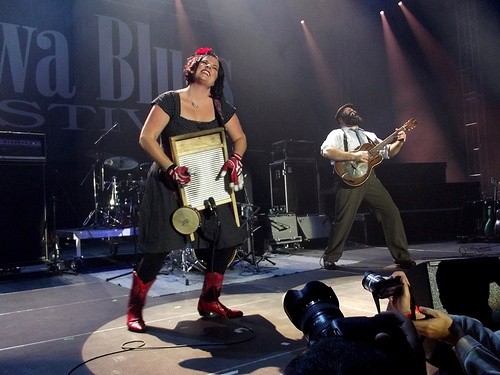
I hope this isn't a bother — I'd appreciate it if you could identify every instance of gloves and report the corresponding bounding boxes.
[217,153,246,192]
[166,164,191,186]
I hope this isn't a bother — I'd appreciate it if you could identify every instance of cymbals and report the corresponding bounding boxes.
[81,149,114,161]
[103,156,139,171]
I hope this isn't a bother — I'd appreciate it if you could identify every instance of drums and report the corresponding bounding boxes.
[97,177,123,211]
[118,190,141,226]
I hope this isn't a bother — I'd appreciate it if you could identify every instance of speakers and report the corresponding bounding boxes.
[0,163,49,266]
[267,212,333,249]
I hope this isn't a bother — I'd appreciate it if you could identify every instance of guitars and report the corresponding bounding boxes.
[483,176,500,238]
[332,117,417,190]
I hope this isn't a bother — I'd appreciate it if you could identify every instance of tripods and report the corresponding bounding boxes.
[229,210,274,273]
[79,159,123,232]
[164,249,206,286]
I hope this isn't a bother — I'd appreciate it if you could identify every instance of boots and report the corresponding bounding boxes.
[196,272,244,318]
[127,269,158,332]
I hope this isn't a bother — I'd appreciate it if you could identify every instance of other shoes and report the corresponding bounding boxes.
[323,257,336,270]
[393,258,417,268]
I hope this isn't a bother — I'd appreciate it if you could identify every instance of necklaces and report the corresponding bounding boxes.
[185,90,200,108]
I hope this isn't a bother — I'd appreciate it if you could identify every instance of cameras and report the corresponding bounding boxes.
[282,280,396,349]
[361,272,403,300]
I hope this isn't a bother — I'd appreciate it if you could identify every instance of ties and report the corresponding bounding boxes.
[354,127,365,146]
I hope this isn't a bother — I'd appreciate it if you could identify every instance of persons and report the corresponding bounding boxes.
[319,104,416,270]
[284,271,500,375]
[126,47,248,332]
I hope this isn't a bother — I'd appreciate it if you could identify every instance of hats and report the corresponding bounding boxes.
[335,103,356,119]
